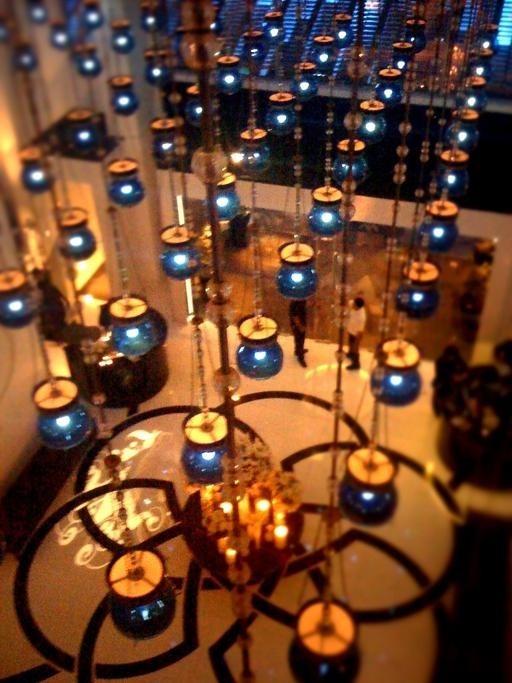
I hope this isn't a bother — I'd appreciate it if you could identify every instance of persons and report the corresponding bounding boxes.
[430,343,471,419]
[32,263,72,345]
[289,299,311,367]
[342,297,368,372]
[453,375,489,435]
